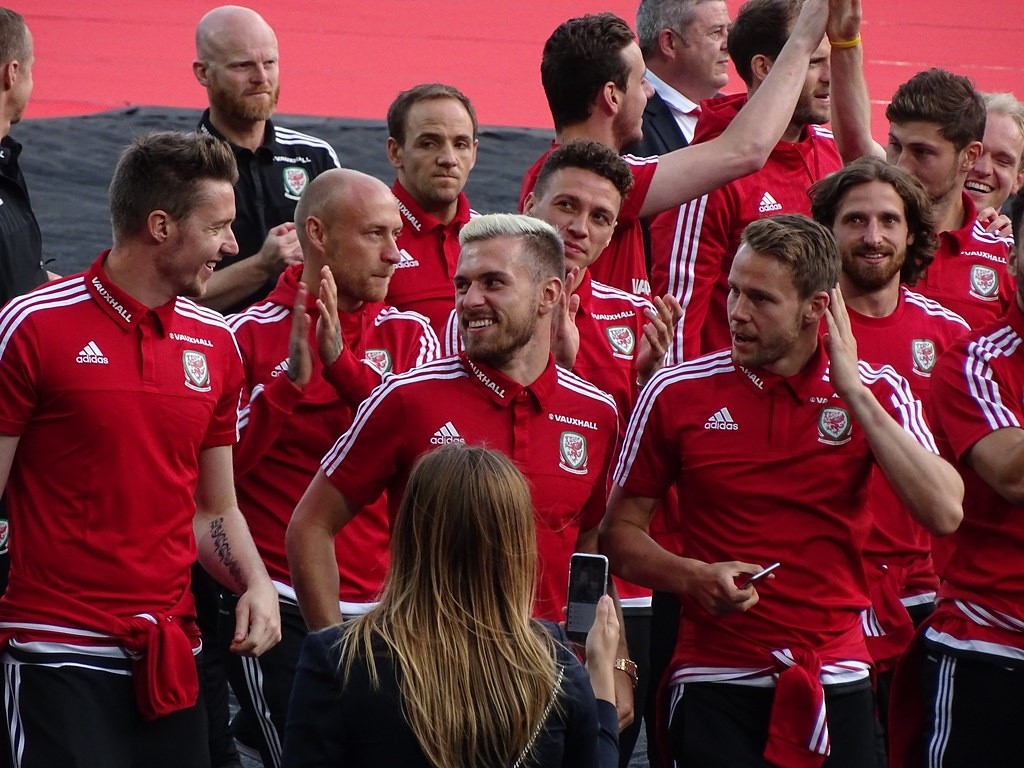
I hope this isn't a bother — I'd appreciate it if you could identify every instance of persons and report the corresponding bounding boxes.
[0,0,1024,768]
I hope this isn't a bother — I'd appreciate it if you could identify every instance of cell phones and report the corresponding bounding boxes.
[738,562,780,590]
[565,553,608,643]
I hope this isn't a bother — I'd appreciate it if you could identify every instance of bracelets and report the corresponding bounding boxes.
[829,33,862,48]
[636,377,645,387]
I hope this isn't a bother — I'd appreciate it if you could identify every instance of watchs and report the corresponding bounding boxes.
[614,658,638,688]
